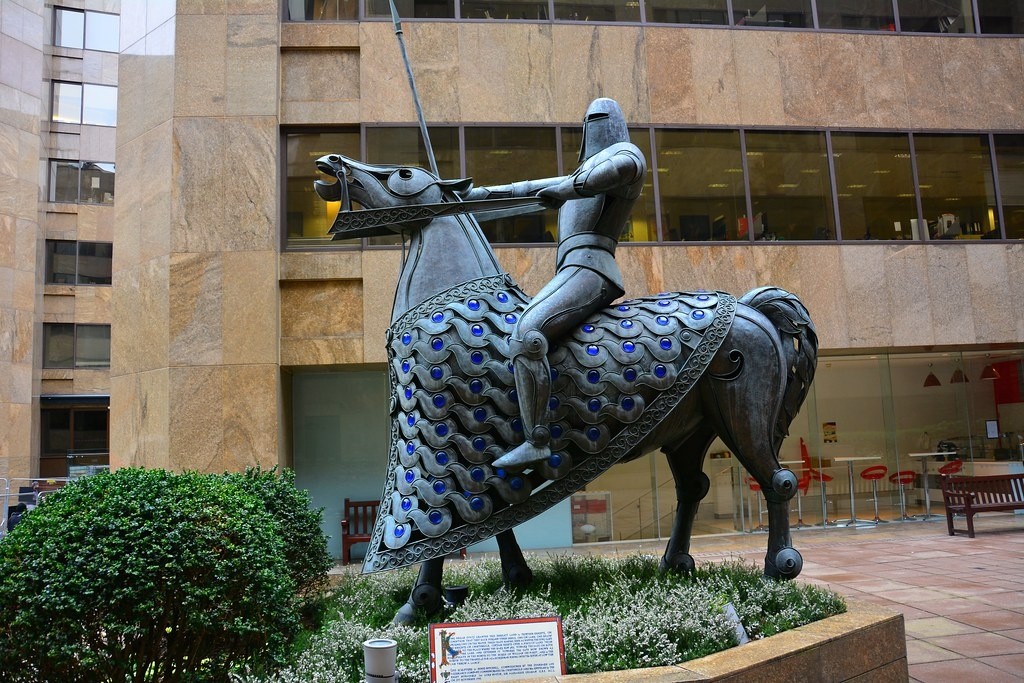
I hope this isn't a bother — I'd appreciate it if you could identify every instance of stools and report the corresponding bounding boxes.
[744,477,768,531]
[939,460,962,477]
[789,475,810,529]
[860,466,888,523]
[889,470,916,521]
[811,469,837,527]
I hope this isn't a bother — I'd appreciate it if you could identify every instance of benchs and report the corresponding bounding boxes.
[942,473,1024,538]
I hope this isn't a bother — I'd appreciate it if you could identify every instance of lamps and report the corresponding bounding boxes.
[980,353,1001,380]
[924,363,942,387]
[950,358,970,383]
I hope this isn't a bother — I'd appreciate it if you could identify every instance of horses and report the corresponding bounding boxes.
[313,154,820,633]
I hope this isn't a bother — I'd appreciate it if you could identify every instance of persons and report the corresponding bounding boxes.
[460,96,646,467]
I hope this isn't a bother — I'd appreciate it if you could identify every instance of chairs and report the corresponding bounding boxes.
[340,497,381,564]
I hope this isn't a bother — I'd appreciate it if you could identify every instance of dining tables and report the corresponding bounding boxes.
[746,19,786,26]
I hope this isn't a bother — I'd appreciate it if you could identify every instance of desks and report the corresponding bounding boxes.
[571,489,612,540]
[907,452,956,519]
[778,460,805,464]
[834,456,882,526]
